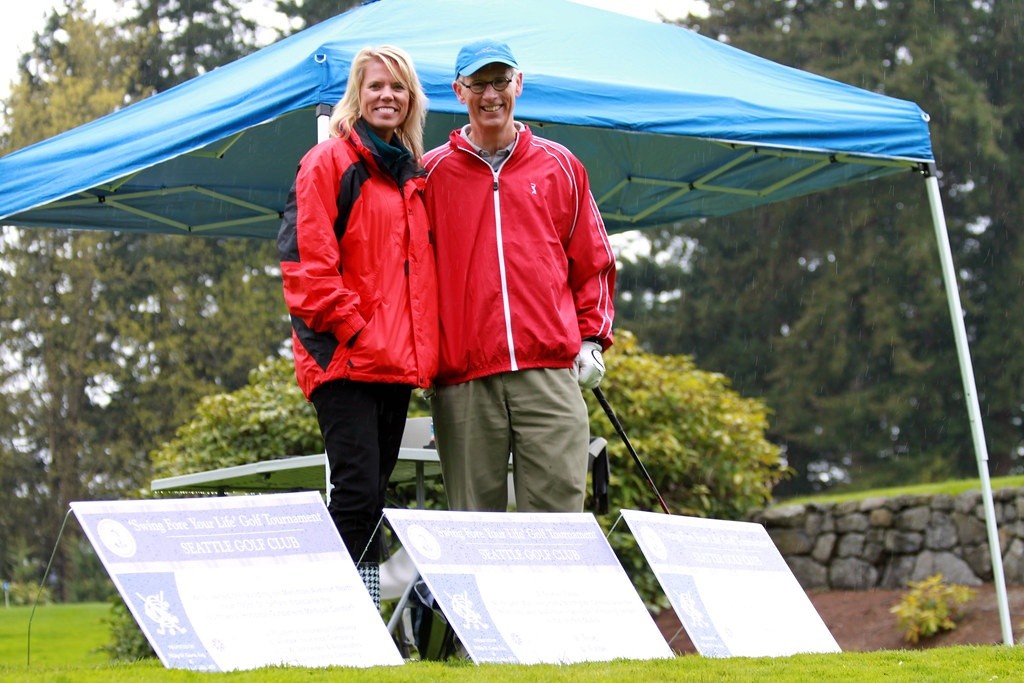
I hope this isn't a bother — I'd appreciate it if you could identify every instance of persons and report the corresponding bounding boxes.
[275,41,440,615]
[411,41,618,514]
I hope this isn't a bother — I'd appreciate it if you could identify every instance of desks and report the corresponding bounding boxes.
[149,447,442,640]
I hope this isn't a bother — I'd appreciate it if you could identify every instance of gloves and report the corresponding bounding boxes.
[579,341,605,388]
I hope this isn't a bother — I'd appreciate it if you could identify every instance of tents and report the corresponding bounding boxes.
[0,0,1016,649]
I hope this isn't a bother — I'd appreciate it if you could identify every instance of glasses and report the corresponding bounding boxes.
[459,75,514,95]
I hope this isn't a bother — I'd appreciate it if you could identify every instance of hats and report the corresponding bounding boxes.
[454,39,519,82]
